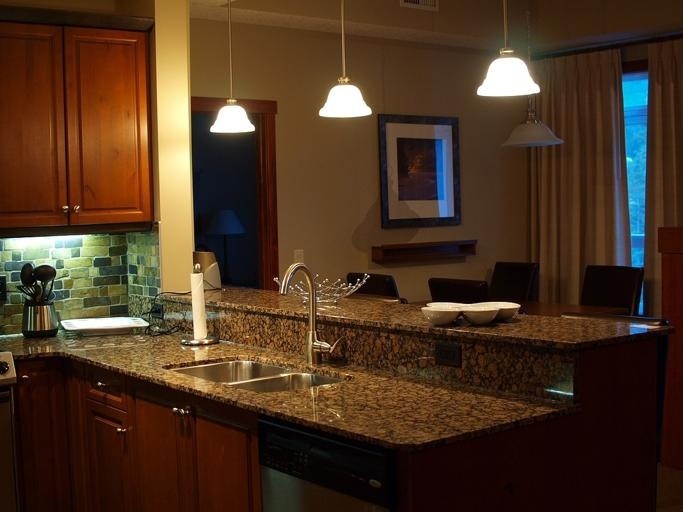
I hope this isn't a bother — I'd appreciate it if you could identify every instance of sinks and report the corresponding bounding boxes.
[160,350,295,387]
[222,364,353,395]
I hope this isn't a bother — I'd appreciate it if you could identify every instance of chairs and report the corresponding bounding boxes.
[491,262,539,303]
[428,278,490,304]
[347,272,399,298]
[579,265,643,316]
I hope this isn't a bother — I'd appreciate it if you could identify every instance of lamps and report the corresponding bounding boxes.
[476,0,541,97]
[206,208,247,282]
[316,0,372,119]
[502,10,565,149]
[209,1,256,135]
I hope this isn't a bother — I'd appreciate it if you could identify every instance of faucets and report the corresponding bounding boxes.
[273,261,346,367]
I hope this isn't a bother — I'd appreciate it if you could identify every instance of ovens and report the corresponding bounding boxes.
[258,419,396,512]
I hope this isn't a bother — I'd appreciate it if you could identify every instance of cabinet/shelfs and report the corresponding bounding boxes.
[129,379,261,511]
[69,360,129,511]
[1,13,154,228]
[17,359,69,511]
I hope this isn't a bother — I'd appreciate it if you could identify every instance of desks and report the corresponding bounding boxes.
[409,298,627,316]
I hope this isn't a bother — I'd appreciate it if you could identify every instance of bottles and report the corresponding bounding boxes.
[21,302,58,338]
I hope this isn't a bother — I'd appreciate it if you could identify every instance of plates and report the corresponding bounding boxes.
[60,316,149,335]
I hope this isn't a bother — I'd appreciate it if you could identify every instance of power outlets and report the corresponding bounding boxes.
[435,343,461,368]
[152,304,163,318]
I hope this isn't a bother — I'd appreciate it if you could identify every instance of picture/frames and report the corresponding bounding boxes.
[378,114,462,229]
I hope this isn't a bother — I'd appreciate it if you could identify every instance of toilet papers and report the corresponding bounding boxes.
[190,270,209,340]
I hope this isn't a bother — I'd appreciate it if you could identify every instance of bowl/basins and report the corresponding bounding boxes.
[420,302,520,327]
